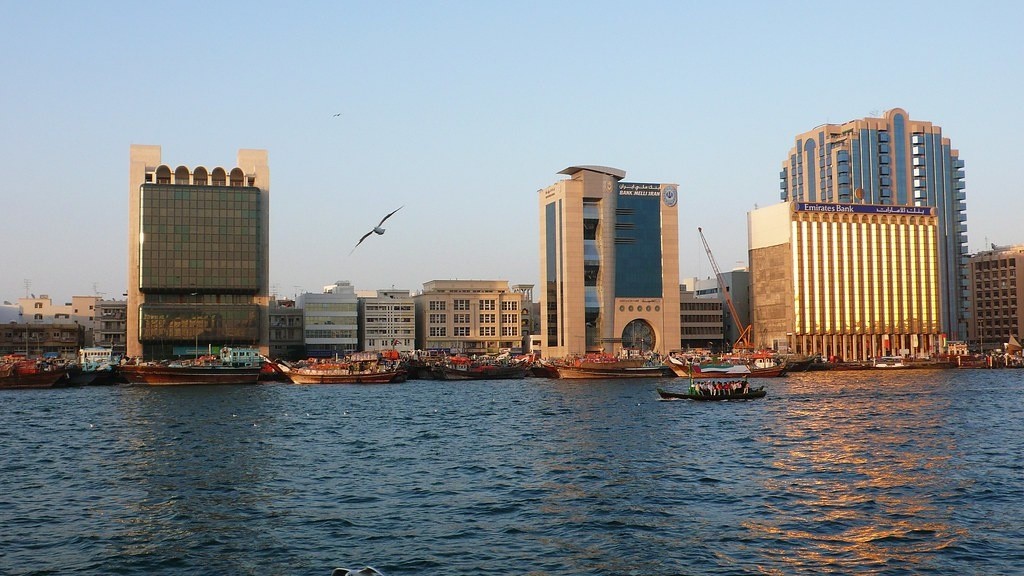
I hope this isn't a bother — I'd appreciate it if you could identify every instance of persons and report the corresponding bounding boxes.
[643,357,653,368]
[904,353,918,360]
[5,355,59,372]
[688,379,751,396]
[598,356,610,364]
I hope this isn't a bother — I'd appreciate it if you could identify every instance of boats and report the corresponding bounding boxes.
[868,362,911,370]
[655,379,768,400]
[0,348,816,384]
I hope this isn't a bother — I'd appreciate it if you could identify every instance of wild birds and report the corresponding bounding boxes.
[333,113,342,117]
[355,205,404,247]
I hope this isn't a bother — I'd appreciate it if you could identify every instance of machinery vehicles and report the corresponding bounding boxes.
[697,226,757,350]
